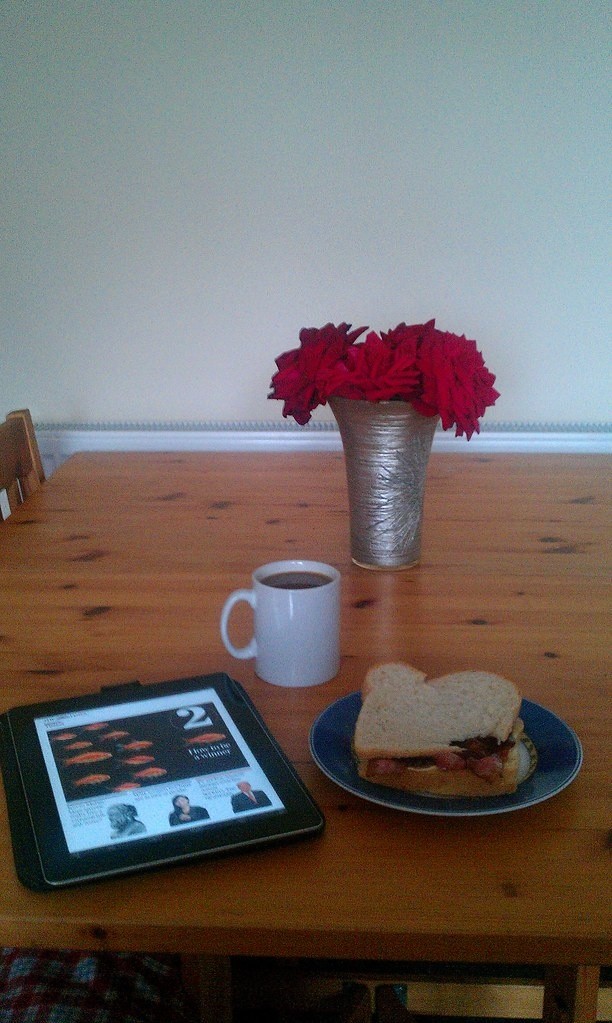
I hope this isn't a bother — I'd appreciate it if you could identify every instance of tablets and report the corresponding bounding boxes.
[1,672,325,889]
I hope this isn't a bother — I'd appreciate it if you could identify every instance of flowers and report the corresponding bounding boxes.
[266,321,501,440]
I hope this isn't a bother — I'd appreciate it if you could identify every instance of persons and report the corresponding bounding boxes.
[169,794,209,826]
[231,781,272,813]
[107,803,148,840]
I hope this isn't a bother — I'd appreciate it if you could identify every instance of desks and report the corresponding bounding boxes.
[0,450,612,1023]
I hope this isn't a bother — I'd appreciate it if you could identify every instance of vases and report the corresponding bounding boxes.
[330,397,443,573]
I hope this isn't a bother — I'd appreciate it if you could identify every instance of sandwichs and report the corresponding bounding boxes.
[351,662,525,798]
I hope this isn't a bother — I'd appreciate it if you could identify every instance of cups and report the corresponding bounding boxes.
[220,560,339,687]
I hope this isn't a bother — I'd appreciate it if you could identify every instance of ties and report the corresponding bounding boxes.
[248,792,257,803]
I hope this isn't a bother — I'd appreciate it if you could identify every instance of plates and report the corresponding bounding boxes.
[308,692,583,816]
